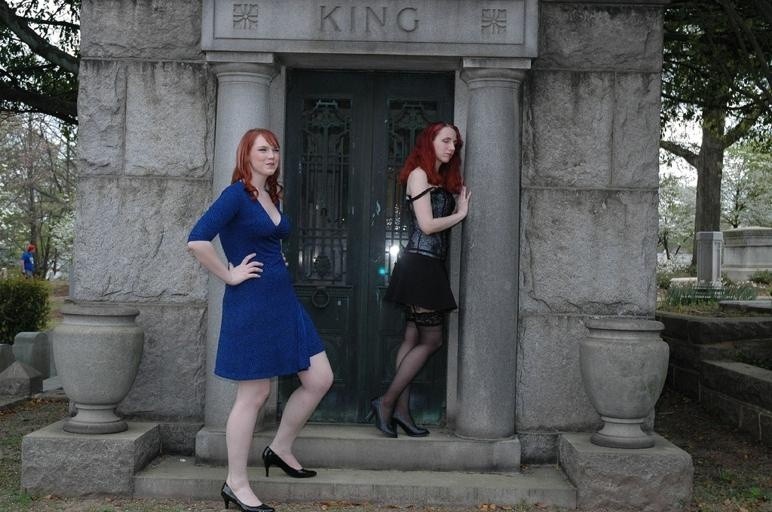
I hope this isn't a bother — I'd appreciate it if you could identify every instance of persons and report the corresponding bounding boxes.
[366,120,472,438]
[20,244,36,281]
[187,127,334,512]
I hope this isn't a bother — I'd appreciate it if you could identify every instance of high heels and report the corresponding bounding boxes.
[365,398,430,438]
[262,446,316,478]
[221,481,274,512]
